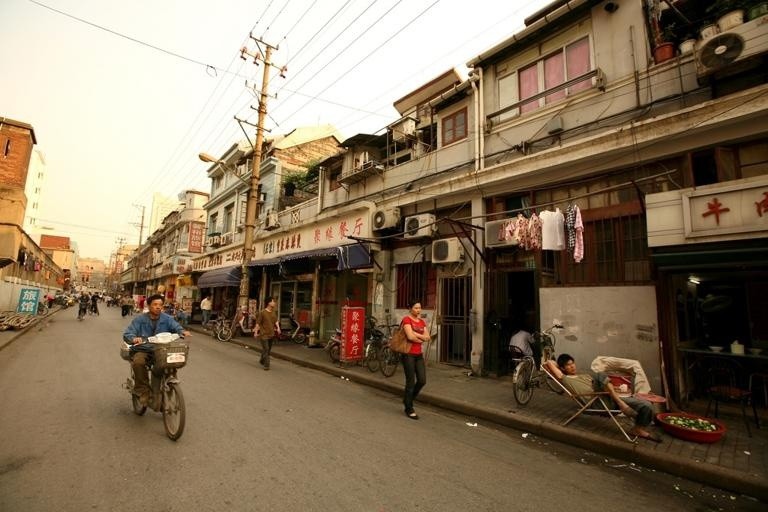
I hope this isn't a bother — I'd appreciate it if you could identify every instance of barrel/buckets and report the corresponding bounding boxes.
[644,432,662,443]
[622,406,638,417]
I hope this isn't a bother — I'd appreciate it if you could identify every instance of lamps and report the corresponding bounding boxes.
[704,372,760,438]
[537,360,638,443]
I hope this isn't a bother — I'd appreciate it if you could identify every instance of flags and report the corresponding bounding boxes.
[653,0,768,64]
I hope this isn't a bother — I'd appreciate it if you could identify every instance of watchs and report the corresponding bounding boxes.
[206,310,257,342]
[120,333,193,440]
[325,328,341,363]
[274,312,306,344]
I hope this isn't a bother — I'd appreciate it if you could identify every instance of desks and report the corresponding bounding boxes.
[199,152,261,310]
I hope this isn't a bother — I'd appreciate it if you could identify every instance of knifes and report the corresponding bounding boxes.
[262,211,279,230]
[371,207,522,264]
[697,13,768,76]
[208,236,220,247]
[392,119,416,141]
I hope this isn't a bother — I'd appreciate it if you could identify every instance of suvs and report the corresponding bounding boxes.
[605,370,633,397]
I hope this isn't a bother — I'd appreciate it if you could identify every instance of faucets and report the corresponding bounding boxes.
[404,408,418,420]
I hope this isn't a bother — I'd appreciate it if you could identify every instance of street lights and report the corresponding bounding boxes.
[391,320,413,354]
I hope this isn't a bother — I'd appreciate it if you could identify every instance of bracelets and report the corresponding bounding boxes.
[748,348,762,355]
[709,346,724,352]
[656,412,726,443]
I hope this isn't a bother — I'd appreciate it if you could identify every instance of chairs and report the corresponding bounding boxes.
[264,366,269,371]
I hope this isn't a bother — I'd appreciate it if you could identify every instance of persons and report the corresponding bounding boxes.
[389,299,431,420]
[545,352,664,442]
[122,294,192,404]
[253,296,282,371]
[509,328,536,356]
[42,284,214,331]
